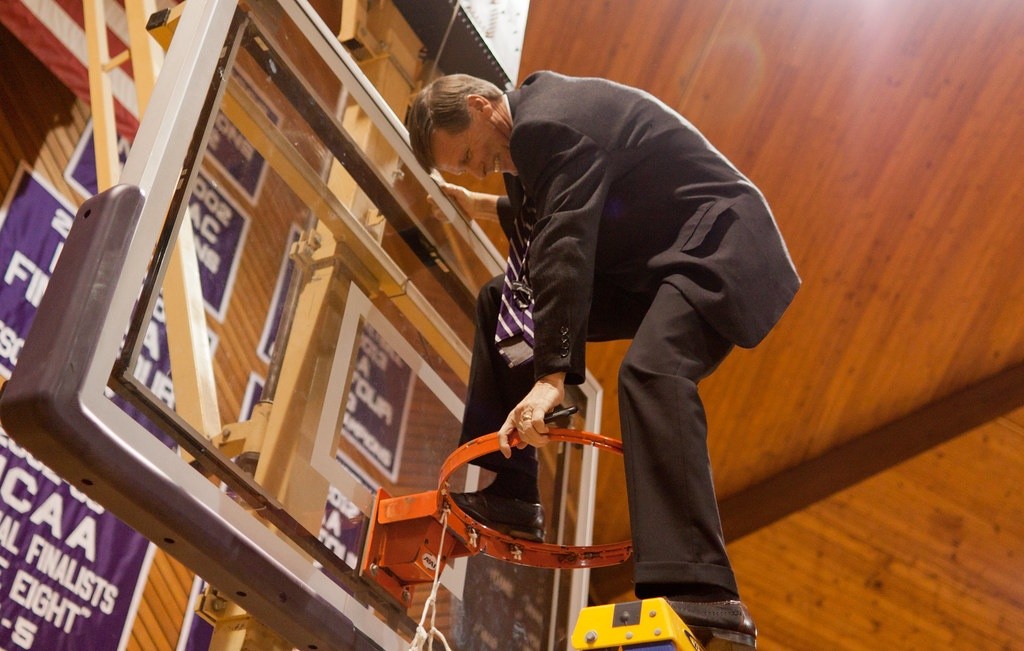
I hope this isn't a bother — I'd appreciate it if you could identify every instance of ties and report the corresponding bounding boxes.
[494,186,538,369]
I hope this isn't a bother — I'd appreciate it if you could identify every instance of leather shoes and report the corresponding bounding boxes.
[449,486,546,542]
[662,596,757,650]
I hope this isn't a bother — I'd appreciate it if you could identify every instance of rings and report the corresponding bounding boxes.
[522,409,533,421]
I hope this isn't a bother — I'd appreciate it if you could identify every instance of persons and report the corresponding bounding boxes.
[407,70,803,651]
